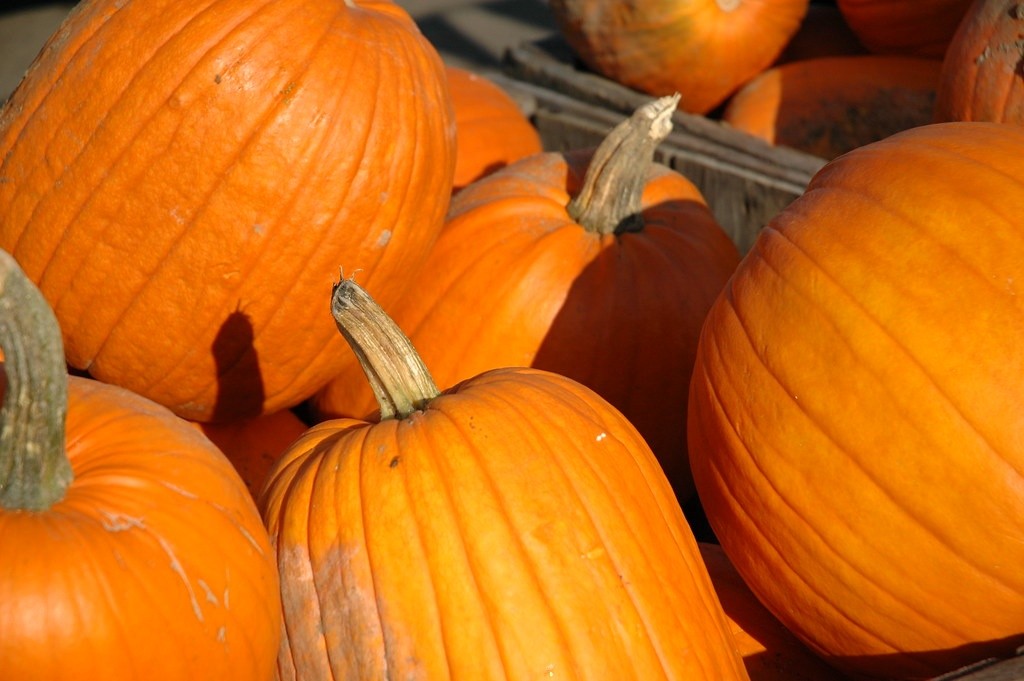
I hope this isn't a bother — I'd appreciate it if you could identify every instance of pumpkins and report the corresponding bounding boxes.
[0,0,1024,681]
